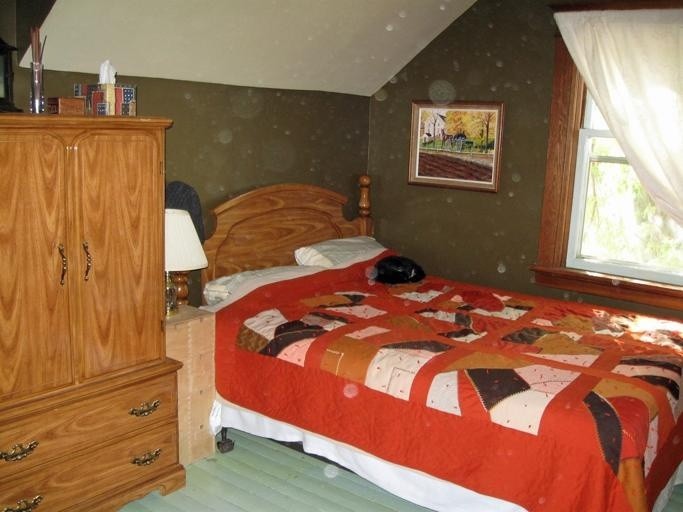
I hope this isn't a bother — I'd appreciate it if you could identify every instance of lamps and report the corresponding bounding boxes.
[164,208,209,318]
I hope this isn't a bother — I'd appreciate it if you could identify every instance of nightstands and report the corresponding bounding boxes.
[165,307,216,465]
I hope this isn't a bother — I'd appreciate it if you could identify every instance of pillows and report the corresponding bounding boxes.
[294,236,385,270]
[203,264,314,304]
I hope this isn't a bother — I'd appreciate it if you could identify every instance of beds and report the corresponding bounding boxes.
[200,174,683,512]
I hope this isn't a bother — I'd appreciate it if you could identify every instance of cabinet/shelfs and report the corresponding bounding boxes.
[0,112,186,512]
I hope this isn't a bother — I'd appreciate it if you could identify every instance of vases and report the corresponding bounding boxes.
[30,63,45,114]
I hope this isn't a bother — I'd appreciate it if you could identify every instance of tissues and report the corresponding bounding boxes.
[72,59,138,118]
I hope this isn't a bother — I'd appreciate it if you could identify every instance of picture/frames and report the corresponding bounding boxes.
[409,100,506,193]
